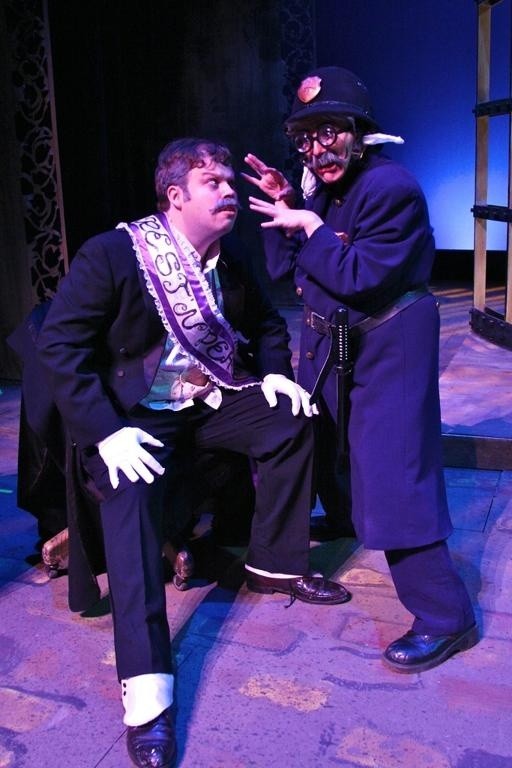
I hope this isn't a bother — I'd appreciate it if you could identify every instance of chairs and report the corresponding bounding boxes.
[17,303,318,590]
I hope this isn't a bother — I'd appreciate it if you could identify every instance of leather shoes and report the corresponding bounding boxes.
[378,621,482,675]
[241,565,350,610]
[306,513,360,542]
[124,708,180,768]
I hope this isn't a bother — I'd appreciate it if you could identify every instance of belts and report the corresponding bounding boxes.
[303,283,434,341]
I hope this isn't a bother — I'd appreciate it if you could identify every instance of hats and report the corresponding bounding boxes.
[284,66,385,155]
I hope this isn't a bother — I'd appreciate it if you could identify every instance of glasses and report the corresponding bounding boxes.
[287,124,348,155]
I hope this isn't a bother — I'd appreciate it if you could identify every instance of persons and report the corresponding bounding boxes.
[31,136,354,767]
[241,65,478,675]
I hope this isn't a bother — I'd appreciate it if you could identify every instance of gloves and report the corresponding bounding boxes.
[260,373,320,417]
[95,426,167,489]
